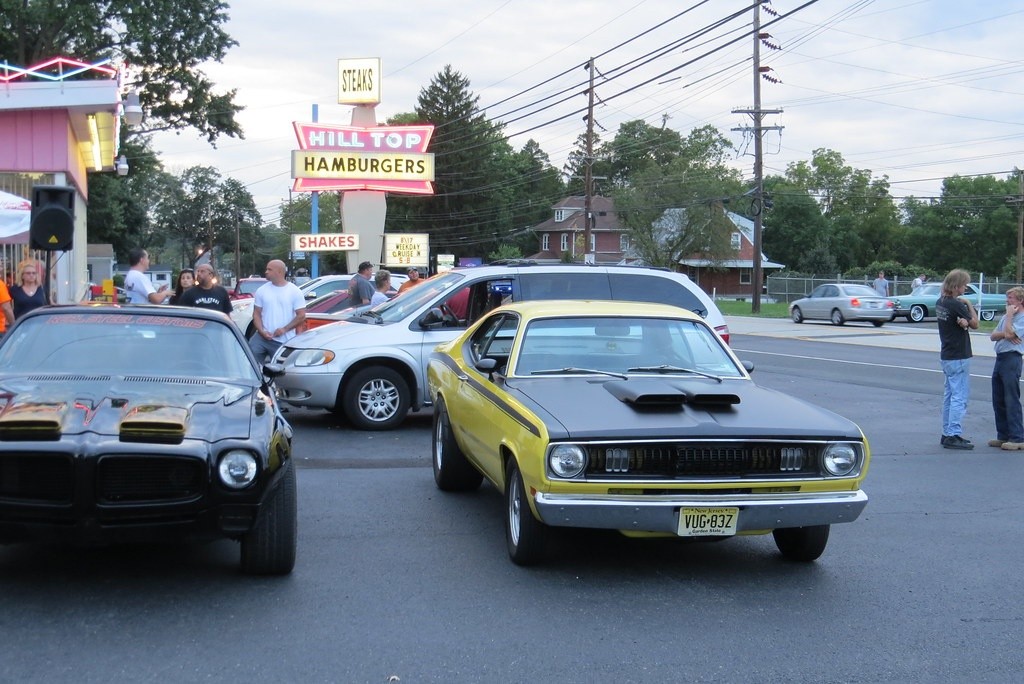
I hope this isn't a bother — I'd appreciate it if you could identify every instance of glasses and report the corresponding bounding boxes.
[182,268,193,271]
[23,271,36,275]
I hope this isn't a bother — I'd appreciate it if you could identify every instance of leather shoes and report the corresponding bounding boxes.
[941,435,971,445]
[943,436,974,450]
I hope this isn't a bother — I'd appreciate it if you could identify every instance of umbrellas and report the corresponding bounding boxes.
[0,192,31,243]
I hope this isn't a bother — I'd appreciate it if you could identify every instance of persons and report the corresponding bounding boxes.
[399,267,425,293]
[10,264,47,320]
[0,279,15,337]
[178,264,233,318]
[446,288,470,320]
[989,287,1024,450]
[911,275,925,291]
[124,247,176,305]
[873,273,889,297]
[371,270,390,309]
[936,269,980,450]
[350,261,375,305]
[169,269,196,305]
[248,260,307,364]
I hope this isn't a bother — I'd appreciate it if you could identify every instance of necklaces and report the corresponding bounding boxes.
[30,292,33,294]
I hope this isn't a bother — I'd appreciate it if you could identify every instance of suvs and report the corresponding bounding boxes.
[269,258,734,431]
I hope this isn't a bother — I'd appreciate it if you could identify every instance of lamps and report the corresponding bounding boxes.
[116,92,143,124]
[115,155,129,175]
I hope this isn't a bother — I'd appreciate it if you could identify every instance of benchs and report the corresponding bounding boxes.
[484,353,639,377]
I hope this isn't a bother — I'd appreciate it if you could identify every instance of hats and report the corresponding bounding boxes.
[406,267,417,273]
[359,262,375,270]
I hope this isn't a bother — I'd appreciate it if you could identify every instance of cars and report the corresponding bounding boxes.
[883,281,1010,322]
[220,272,411,341]
[788,283,896,327]
[426,298,872,569]
[0,302,303,579]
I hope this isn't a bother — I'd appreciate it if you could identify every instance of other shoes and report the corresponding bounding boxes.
[988,440,1006,447]
[1001,442,1024,450]
